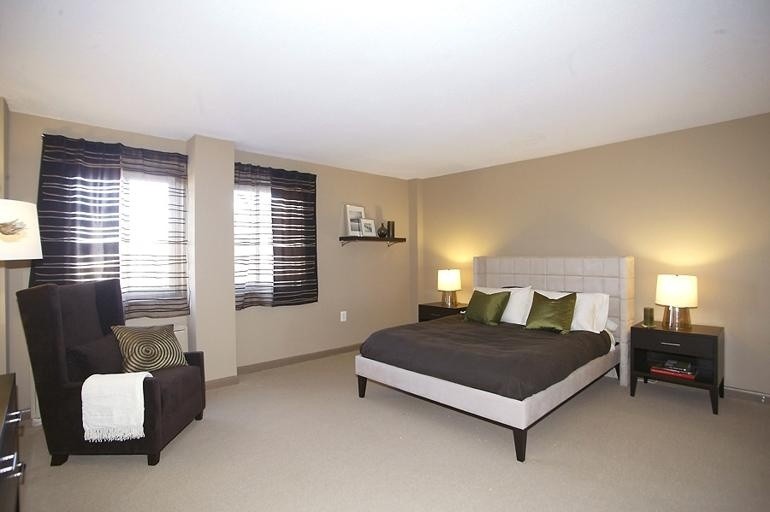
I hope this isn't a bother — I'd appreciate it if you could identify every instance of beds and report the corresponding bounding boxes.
[355,256,634,463]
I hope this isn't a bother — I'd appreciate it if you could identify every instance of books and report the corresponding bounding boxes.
[650,358,699,380]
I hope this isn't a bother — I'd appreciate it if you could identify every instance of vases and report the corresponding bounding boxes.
[388,221,394,238]
[378,223,388,238]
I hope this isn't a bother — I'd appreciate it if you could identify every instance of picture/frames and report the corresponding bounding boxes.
[346,205,365,236]
[361,218,376,237]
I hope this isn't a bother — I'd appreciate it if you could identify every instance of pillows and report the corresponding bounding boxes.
[110,324,189,373]
[462,285,610,336]
[67,335,122,380]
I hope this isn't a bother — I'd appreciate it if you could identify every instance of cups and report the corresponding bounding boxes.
[644,308,654,326]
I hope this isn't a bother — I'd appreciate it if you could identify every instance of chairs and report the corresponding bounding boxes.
[16,279,205,466]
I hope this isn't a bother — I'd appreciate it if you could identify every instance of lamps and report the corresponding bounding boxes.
[437,269,462,305]
[0,199,44,261]
[655,274,699,331]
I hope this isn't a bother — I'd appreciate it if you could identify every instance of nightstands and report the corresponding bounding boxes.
[418,302,468,322]
[631,321,724,414]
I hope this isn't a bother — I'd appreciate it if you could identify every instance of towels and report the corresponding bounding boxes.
[81,371,153,444]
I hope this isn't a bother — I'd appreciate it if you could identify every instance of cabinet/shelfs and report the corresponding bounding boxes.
[0,373,24,512]
